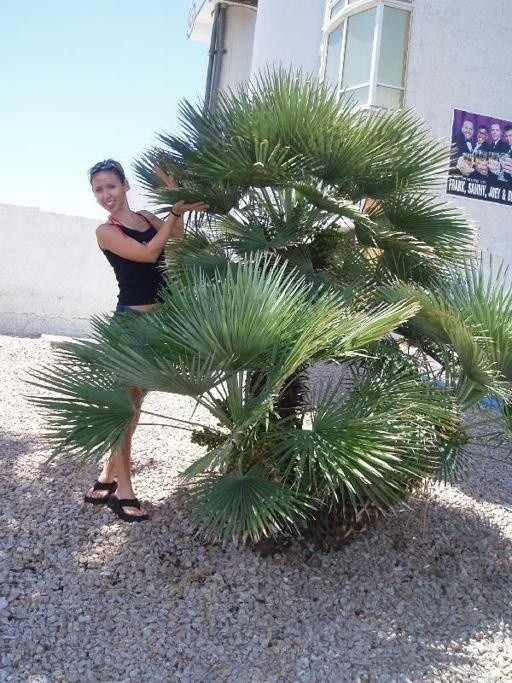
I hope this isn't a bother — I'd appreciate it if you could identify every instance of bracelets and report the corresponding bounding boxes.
[170,209,183,218]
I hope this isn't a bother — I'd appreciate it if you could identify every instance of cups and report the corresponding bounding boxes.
[461,149,509,174]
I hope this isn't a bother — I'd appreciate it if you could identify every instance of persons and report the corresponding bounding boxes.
[85,158,211,522]
[454,119,512,183]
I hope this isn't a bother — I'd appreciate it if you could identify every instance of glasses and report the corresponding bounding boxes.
[90,162,125,179]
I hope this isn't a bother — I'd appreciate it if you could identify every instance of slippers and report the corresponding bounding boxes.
[107,495,149,523]
[85,478,118,504]
